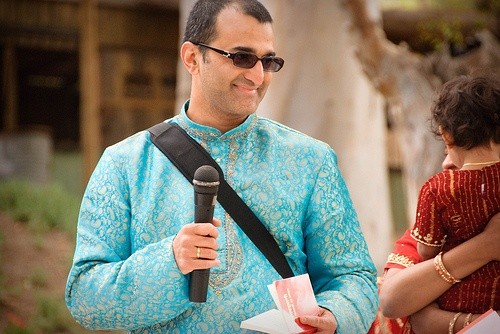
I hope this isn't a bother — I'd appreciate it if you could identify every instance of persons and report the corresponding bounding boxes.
[64,0,380,334]
[369,75,500,334]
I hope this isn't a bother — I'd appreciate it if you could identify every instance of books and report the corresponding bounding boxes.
[240,273,320,334]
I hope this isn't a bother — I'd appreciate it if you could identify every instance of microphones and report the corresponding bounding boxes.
[189,165,220,303]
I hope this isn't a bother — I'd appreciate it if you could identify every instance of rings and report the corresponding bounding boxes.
[197,248,200,258]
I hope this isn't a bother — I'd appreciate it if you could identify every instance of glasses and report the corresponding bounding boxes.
[192,42,284,73]
